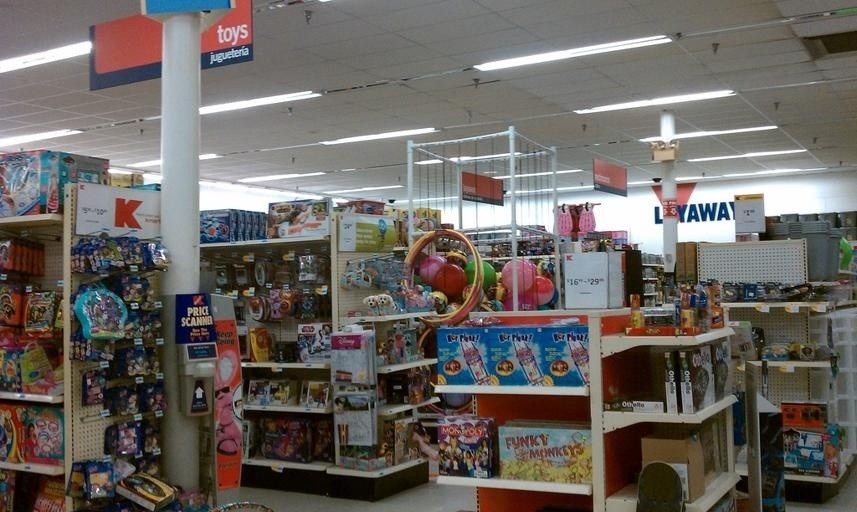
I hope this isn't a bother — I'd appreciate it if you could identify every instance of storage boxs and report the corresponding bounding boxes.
[1,150,145,215]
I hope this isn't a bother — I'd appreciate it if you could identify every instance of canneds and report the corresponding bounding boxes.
[679,309,693,329]
[710,306,725,328]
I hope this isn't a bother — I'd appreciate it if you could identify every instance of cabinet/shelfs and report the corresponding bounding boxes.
[0,181,857,512]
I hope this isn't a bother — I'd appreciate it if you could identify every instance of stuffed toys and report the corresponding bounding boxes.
[363,295,380,315]
[378,294,396,315]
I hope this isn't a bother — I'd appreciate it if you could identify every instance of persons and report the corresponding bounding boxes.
[275,384,287,404]
[412,433,439,461]
[48,175,58,214]
[25,423,39,456]
[443,451,480,477]
